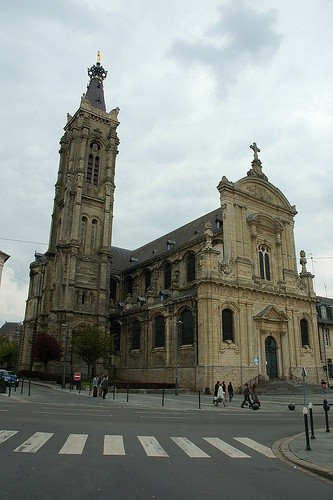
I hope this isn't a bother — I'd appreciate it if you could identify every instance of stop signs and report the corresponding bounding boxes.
[74,373,81,381]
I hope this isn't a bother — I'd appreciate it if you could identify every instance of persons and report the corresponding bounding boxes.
[222,381,228,401]
[215,383,226,407]
[249,384,260,409]
[241,383,253,408]
[92,372,110,399]
[213,381,220,403]
[228,382,233,402]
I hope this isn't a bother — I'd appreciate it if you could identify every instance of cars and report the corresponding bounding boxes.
[0,370,20,388]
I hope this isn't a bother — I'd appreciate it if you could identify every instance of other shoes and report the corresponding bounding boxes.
[224,405,228,407]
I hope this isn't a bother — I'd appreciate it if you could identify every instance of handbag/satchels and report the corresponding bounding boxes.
[216,397,221,402]
